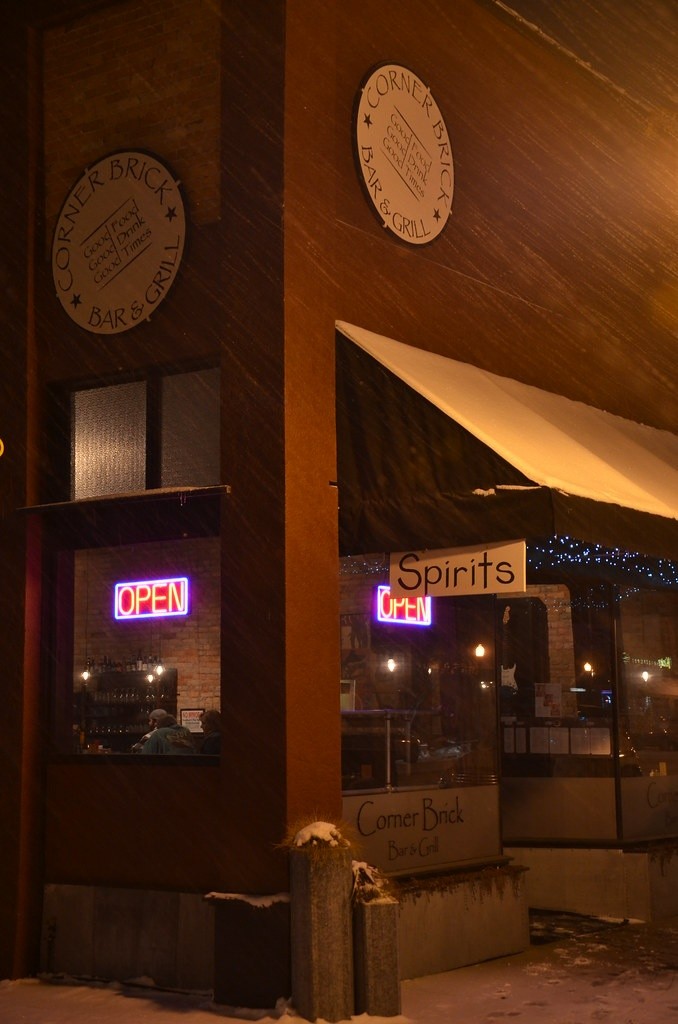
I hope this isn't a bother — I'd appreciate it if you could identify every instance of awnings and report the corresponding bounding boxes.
[335,319,678,520]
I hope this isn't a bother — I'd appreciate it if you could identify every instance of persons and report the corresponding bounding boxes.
[131,709,221,756]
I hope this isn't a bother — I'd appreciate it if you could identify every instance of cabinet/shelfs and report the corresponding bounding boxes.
[83,667,179,742]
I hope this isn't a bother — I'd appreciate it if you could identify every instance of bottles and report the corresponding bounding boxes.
[103,688,155,703]
[86,649,158,672]
